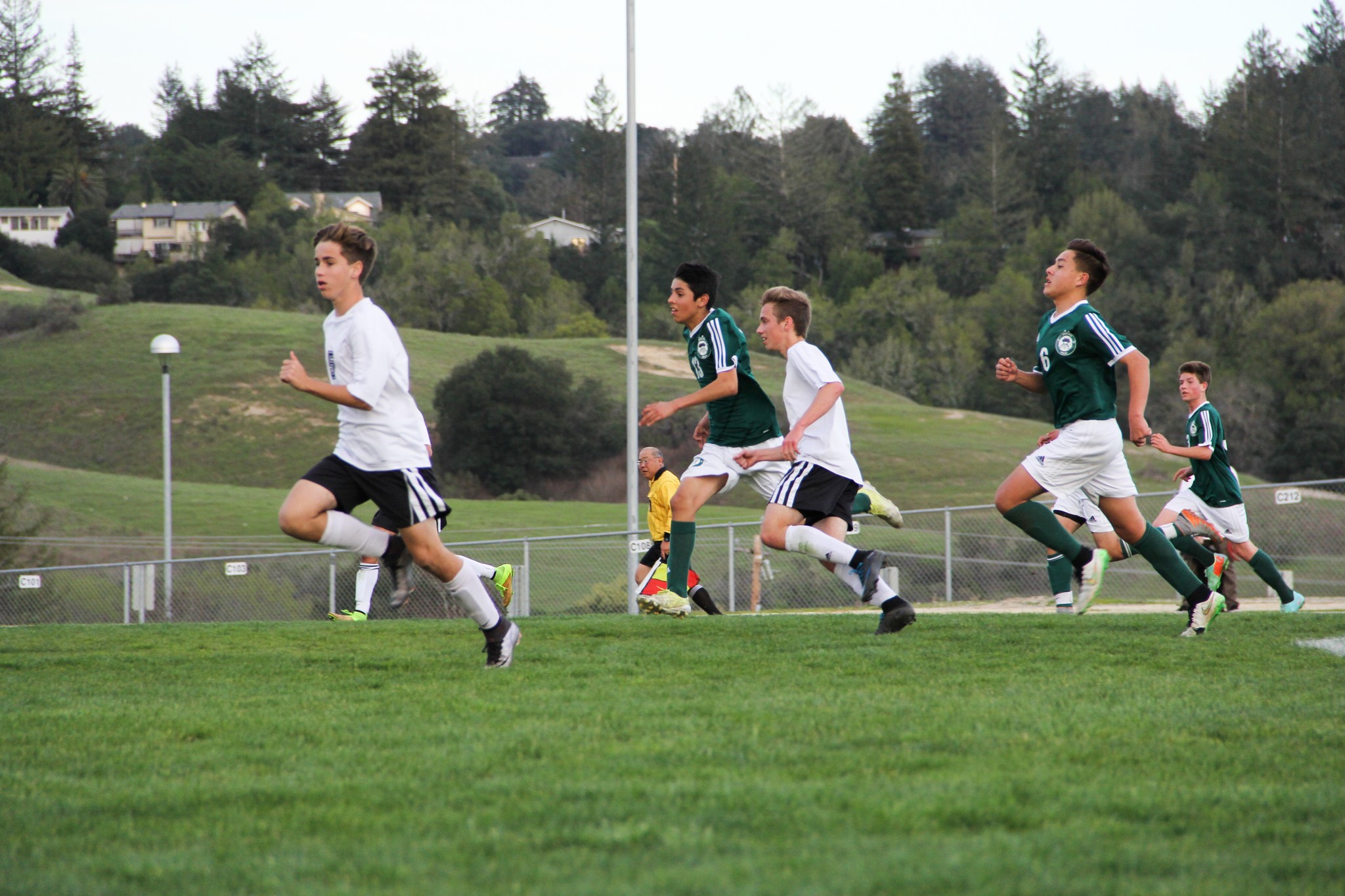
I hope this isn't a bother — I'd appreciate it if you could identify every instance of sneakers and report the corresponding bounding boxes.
[1281,591,1305,613]
[327,609,367,624]
[1174,510,1223,543]
[383,534,414,610]
[492,563,516,608]
[1181,590,1228,637]
[637,586,691,620]
[876,601,916,635]
[1203,552,1229,593]
[481,620,521,669]
[857,480,903,529]
[1072,547,1112,616]
[847,548,887,603]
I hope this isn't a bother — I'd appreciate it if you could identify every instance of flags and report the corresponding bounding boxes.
[636,560,700,596]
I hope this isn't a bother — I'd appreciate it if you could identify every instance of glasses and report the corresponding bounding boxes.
[636,456,660,466]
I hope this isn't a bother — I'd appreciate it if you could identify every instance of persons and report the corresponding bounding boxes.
[1038,431,1225,619]
[733,284,917,634]
[992,239,1225,640]
[327,410,514,622]
[274,222,523,676]
[638,265,904,618]
[635,446,723,617]
[1176,462,1240,614]
[1147,361,1306,615]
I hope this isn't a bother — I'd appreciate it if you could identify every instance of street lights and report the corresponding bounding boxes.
[150,335,182,607]
